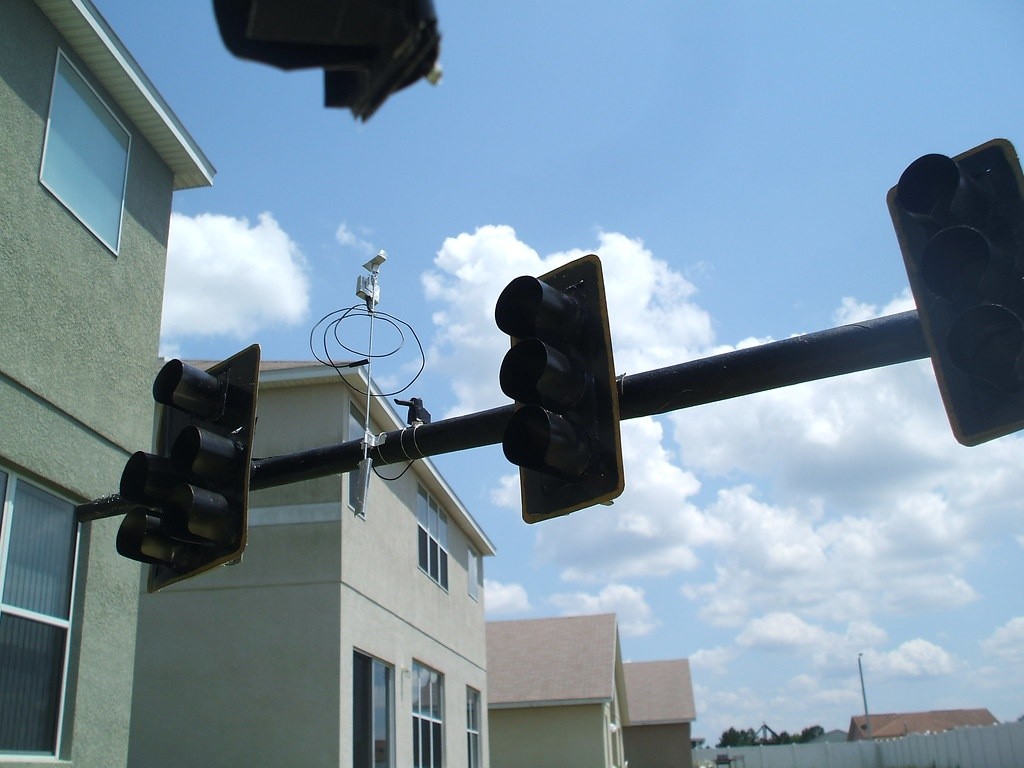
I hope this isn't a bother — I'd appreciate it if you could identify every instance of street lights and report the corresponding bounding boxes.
[858,653,872,740]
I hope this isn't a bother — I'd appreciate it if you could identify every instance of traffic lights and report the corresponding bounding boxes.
[495,254,625,524]
[886,138,1024,448]
[116,342,261,594]
